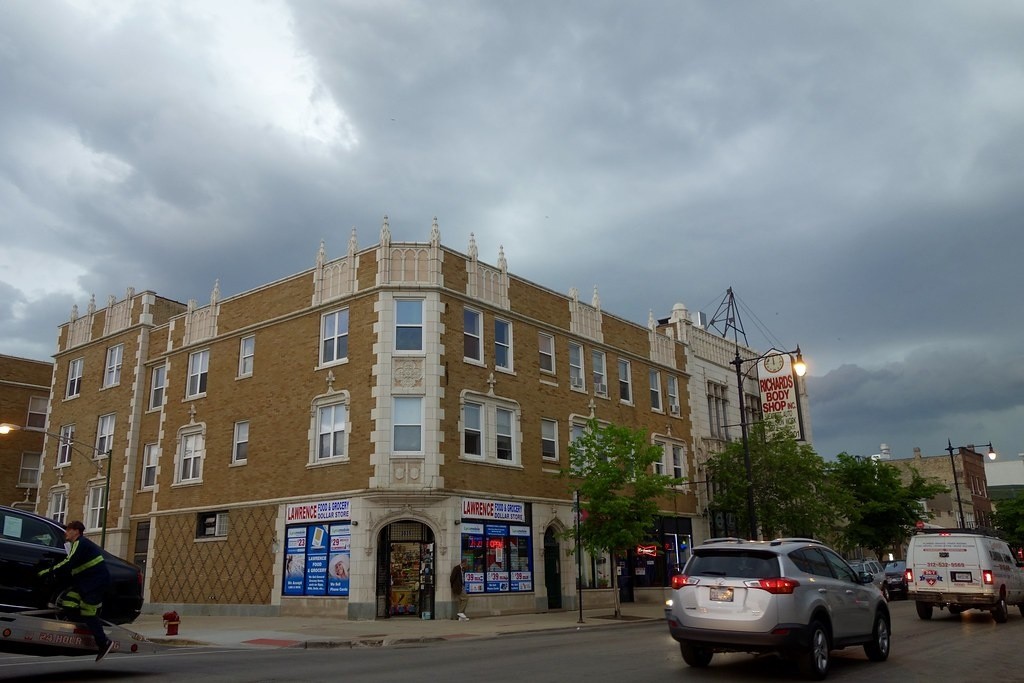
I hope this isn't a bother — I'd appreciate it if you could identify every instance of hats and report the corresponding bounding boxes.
[62,521,85,532]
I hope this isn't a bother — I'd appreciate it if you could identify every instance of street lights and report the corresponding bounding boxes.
[729,343,807,541]
[944,438,997,529]
[0,423,113,550]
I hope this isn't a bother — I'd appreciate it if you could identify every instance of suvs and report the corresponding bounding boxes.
[663,537,891,681]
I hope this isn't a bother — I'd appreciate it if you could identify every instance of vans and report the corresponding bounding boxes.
[844,559,891,603]
[904,521,1024,623]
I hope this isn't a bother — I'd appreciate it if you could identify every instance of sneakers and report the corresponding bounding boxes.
[459,617,469,621]
[457,613,466,619]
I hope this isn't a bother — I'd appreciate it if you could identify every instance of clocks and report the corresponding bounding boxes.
[763,352,783,373]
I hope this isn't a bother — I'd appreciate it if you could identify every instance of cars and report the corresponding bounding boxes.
[0,505,144,627]
[885,560,906,595]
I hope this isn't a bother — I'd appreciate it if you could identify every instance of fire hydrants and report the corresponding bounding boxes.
[162,610,182,636]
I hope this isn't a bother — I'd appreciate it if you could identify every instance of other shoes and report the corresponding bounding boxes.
[95,640,114,663]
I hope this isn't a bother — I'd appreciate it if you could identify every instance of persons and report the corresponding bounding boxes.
[451,559,469,620]
[37,521,114,662]
